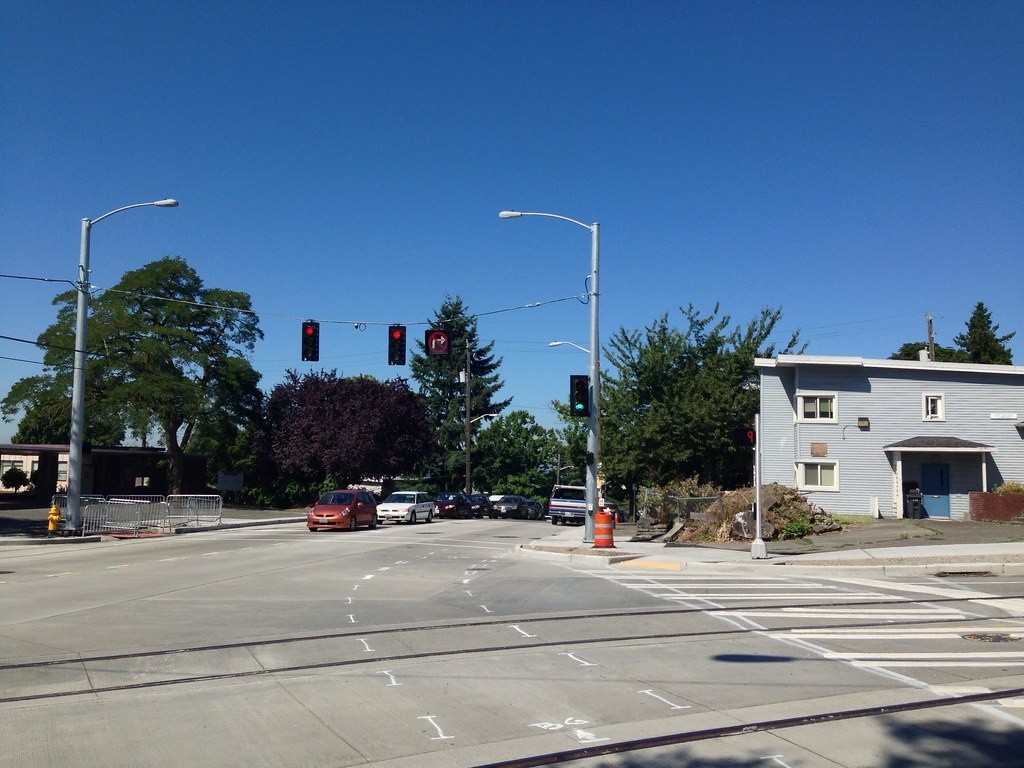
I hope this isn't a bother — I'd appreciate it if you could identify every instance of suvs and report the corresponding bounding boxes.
[548,484,641,526]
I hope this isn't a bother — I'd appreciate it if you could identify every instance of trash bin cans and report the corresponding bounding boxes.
[905,494,922,519]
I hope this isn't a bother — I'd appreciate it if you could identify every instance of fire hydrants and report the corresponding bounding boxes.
[47,504,62,538]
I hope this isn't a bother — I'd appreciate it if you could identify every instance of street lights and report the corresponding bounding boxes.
[557,465,575,485]
[465,412,499,495]
[60,197,180,537]
[498,209,599,542]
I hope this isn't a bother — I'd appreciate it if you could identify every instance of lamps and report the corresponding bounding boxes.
[858,416,871,429]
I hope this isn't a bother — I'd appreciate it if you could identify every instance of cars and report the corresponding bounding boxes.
[432,491,471,519]
[306,489,378,533]
[377,490,435,525]
[465,494,494,519]
[488,493,546,520]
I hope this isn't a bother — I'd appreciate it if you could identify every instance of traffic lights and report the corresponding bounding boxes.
[301,322,319,362]
[388,325,406,366]
[570,375,589,417]
[738,429,757,449]
